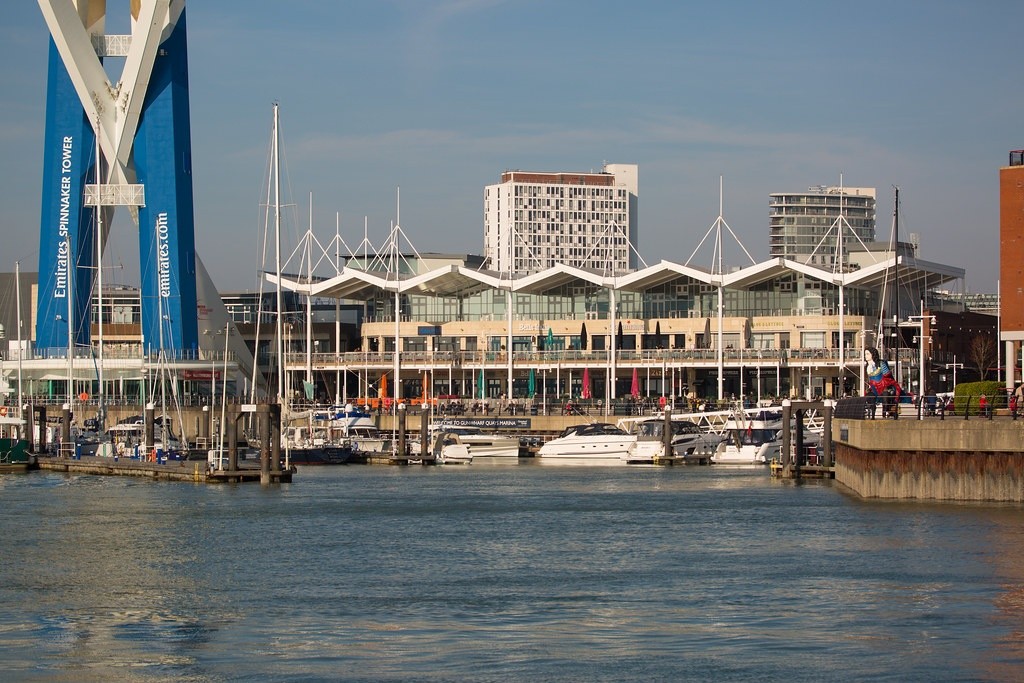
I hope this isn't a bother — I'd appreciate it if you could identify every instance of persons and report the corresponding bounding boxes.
[927,390,938,416]
[289,398,516,417]
[624,395,827,416]
[867,388,876,420]
[864,348,902,420]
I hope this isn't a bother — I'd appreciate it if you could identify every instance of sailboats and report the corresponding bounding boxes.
[1,119,187,467]
[187,101,364,465]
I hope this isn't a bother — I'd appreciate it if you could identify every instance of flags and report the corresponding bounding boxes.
[303,380,314,399]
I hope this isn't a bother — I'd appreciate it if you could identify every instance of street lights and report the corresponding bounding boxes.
[946,363,963,394]
[288,325,293,407]
[873,333,897,360]
[909,315,938,421]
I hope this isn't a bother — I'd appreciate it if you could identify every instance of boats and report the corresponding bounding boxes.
[710,321,819,468]
[537,422,638,460]
[248,402,384,452]
[625,418,725,462]
[406,353,521,457]
[432,431,473,464]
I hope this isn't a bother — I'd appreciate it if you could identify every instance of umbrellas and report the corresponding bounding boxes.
[547,328,554,344]
[421,375,428,398]
[656,321,661,349]
[631,368,639,399]
[704,318,711,348]
[618,322,623,350]
[381,374,387,397]
[580,323,587,349]
[477,370,485,398]
[744,320,751,348]
[528,368,535,398]
[583,368,589,399]
